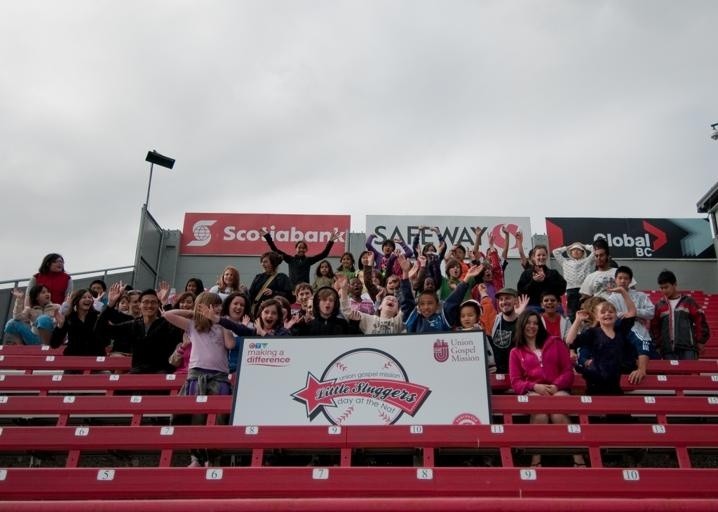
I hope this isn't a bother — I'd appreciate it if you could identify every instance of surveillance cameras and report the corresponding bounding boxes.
[711,129,718,139]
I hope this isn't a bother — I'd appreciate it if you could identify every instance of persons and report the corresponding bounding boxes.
[648,270,710,360]
[165,227,654,468]
[5,253,204,468]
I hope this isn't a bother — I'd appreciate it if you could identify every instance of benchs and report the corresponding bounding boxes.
[0,290,717,511]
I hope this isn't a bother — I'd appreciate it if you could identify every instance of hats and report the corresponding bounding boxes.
[495,288,518,300]
